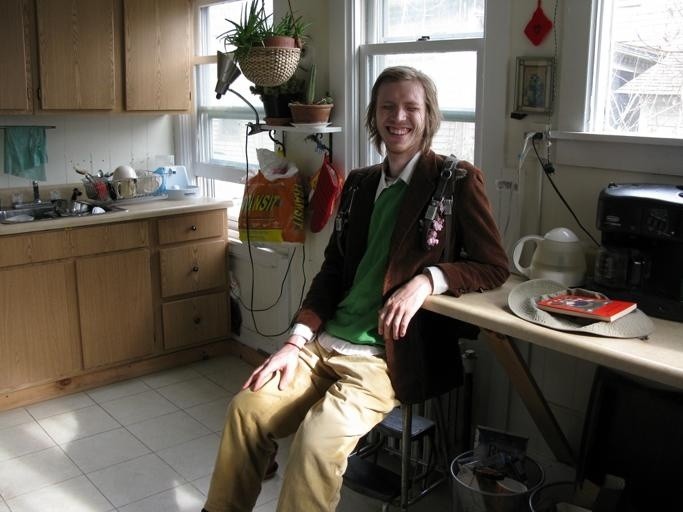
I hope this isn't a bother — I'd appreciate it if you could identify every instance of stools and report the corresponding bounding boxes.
[343,366,458,512]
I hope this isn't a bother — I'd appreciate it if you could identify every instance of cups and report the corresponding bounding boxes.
[116,180,136,199]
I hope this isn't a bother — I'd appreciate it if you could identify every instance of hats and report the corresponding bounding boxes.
[506,280,654,338]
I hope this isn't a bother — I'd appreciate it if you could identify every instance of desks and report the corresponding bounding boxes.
[400,264,682,512]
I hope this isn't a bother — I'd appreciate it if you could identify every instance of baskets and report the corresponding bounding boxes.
[234,48,301,87]
[81,172,171,206]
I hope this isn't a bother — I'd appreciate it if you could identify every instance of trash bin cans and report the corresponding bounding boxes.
[529,480,618,512]
[450,447,545,512]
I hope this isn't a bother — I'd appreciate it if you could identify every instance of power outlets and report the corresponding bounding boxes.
[528,123,550,160]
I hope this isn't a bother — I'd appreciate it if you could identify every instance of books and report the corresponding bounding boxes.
[537,294,638,323]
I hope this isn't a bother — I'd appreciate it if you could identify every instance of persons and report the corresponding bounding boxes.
[199,65,510,512]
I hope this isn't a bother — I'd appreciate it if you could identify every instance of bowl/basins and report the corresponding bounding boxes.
[166,184,200,201]
[53,199,94,217]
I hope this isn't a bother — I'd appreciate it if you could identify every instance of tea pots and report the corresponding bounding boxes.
[508,225,589,290]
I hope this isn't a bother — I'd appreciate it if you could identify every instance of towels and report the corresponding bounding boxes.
[4,126,47,183]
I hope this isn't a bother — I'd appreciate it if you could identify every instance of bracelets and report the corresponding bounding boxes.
[285,342,302,350]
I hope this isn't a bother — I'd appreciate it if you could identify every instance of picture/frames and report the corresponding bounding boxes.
[513,55,554,113]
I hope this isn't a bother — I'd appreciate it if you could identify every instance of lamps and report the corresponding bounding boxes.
[215,50,263,135]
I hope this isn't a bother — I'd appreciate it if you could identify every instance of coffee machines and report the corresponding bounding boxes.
[585,175,683,322]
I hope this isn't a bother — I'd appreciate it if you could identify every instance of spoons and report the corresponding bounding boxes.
[96,170,104,177]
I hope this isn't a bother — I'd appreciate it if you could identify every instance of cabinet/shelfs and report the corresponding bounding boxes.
[0,0,193,116]
[157,209,231,374]
[0,218,157,413]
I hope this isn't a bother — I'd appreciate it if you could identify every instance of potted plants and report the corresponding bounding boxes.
[216,0,313,88]
[249,79,304,117]
[287,63,334,124]
